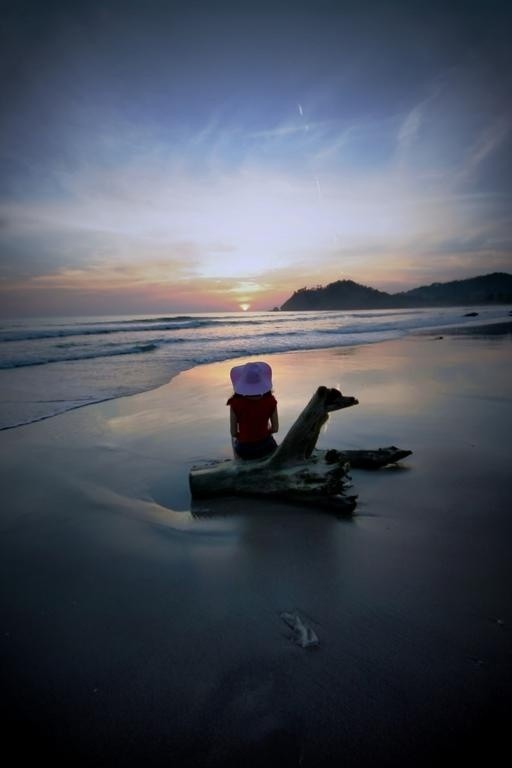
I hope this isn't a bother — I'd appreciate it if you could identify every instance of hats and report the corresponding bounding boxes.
[231,362,272,396]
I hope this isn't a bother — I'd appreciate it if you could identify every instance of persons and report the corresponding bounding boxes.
[228,360,279,461]
